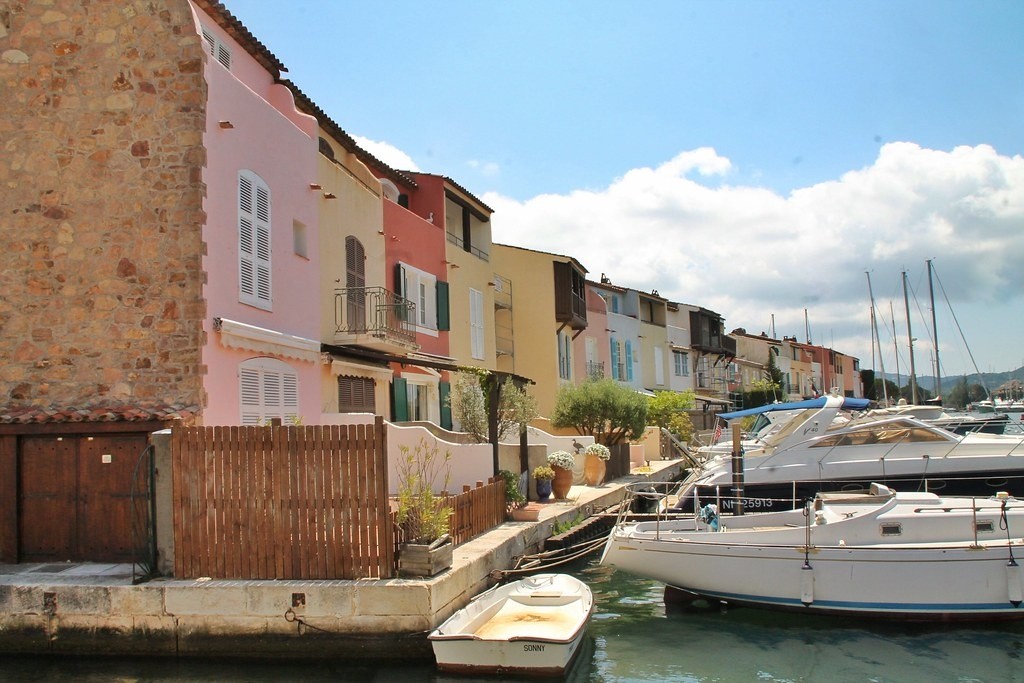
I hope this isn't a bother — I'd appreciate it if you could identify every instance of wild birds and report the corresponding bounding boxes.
[572,439,585,452]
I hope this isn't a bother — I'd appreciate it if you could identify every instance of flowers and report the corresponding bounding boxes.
[584,442,611,461]
[547,449,576,472]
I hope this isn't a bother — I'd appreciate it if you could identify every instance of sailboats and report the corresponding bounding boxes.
[696,259,1009,461]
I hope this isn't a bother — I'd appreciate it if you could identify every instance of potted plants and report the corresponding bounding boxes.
[394,436,453,576]
[532,466,555,500]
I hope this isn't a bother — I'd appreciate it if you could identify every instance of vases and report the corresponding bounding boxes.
[585,454,606,486]
[513,509,540,522]
[551,465,574,501]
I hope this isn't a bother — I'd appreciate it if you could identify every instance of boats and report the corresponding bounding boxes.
[656,387,1024,522]
[971,396,1024,414]
[425,574,592,683]
[599,421,1024,628]
